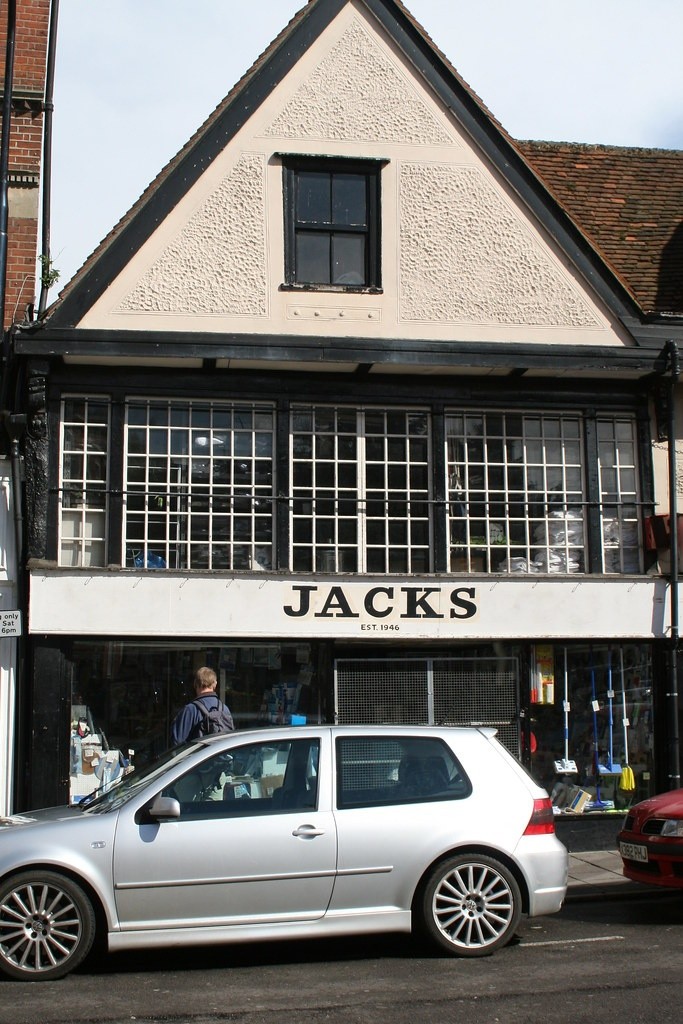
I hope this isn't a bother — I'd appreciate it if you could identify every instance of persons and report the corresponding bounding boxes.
[167,668,234,802]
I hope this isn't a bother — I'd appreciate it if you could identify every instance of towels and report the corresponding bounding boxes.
[498,508,640,574]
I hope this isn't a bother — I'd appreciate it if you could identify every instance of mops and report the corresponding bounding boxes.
[588,644,636,812]
[554,648,578,774]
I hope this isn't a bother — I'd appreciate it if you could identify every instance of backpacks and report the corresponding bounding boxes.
[193,699,234,774]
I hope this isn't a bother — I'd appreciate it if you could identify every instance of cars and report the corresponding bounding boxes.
[615,788,683,888]
[0,725,570,983]
